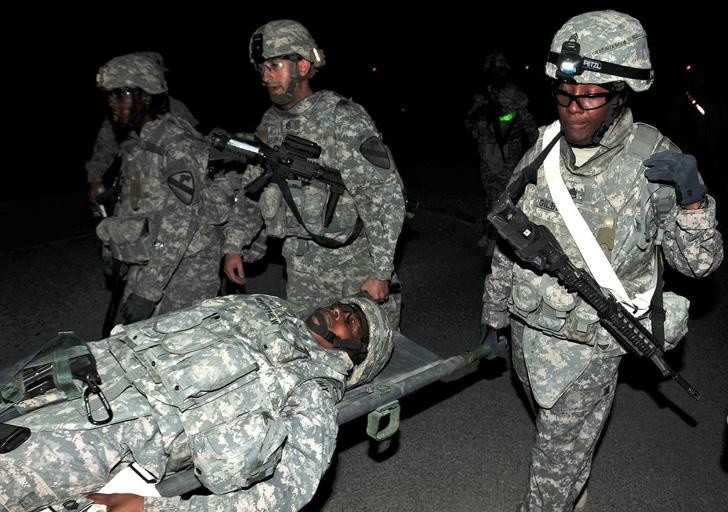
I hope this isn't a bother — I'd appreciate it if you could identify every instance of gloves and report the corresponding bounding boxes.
[643,151,709,206]
[479,325,508,361]
[119,293,156,325]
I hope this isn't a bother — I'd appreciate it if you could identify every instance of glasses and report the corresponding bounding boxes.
[253,60,284,73]
[550,81,619,111]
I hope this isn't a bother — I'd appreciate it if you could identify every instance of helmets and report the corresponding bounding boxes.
[96,52,169,95]
[249,19,326,69]
[484,53,510,71]
[545,9,655,93]
[338,297,395,391]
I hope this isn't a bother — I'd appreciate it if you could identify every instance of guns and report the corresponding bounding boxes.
[487,194,699,400]
[0,337,100,454]
[210,134,422,227]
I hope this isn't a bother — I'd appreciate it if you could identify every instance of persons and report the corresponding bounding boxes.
[223,18,408,334]
[0,292,395,512]
[85,53,225,326]
[464,54,539,259]
[481,9,725,512]
[86,51,200,212]
